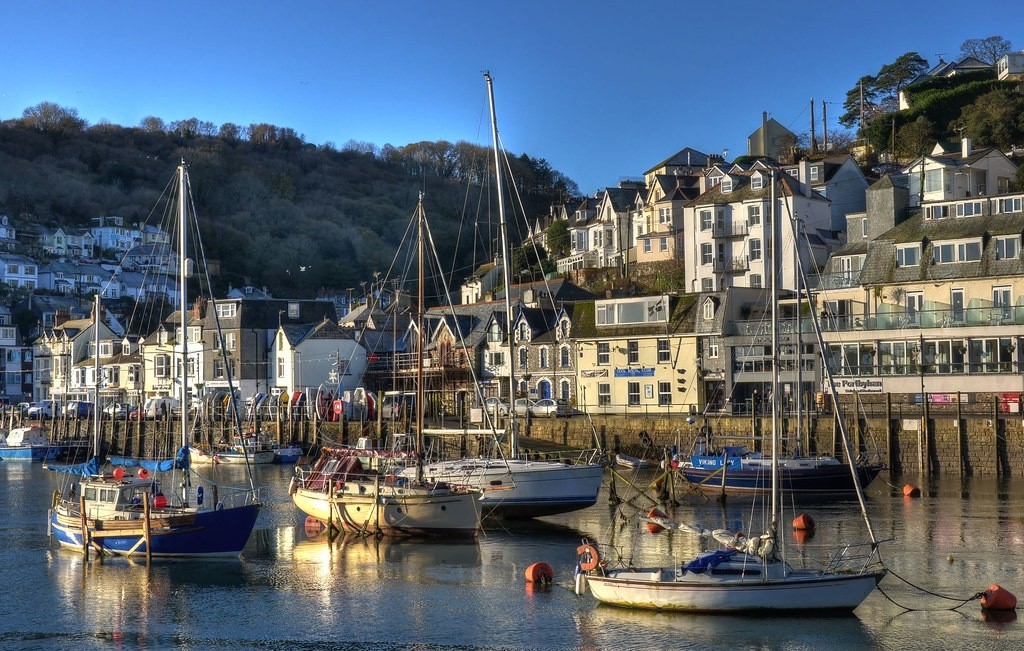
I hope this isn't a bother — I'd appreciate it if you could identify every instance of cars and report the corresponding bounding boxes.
[66,401,109,420]
[482,397,510,415]
[525,399,573,418]
[510,398,541,417]
[103,403,131,419]
[116,406,144,419]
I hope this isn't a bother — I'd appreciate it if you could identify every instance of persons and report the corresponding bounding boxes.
[752,389,774,413]
[821,308,829,333]
[828,308,837,332]
[160,400,167,421]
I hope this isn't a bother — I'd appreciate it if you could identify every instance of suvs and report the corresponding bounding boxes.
[144,396,180,420]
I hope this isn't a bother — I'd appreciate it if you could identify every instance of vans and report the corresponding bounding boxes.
[28,400,62,419]
[381,394,415,417]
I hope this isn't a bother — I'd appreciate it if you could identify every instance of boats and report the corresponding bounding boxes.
[616,453,648,468]
[289,192,516,544]
[400,69,603,511]
[583,170,886,613]
[188,436,303,463]
[48,156,259,557]
[201,387,378,421]
[671,215,886,496]
[0,430,91,462]
